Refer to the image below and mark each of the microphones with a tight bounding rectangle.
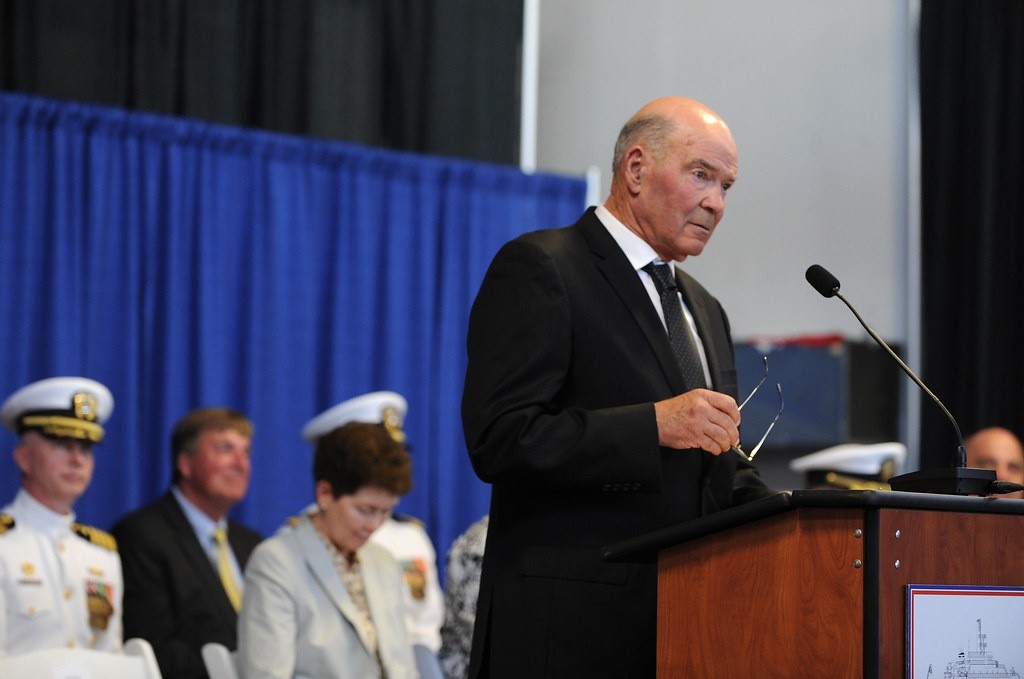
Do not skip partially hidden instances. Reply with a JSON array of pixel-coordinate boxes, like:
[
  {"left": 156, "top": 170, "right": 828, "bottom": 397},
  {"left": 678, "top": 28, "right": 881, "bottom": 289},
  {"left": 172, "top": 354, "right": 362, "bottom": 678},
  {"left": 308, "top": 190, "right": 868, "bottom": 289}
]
[{"left": 805, "top": 264, "right": 998, "bottom": 497}]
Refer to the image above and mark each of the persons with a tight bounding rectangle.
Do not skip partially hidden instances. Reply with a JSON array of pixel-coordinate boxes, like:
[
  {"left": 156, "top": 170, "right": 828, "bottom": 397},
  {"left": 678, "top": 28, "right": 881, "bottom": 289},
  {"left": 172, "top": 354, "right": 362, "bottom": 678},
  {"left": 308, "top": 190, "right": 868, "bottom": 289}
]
[
  {"left": 961, "top": 426, "right": 1024, "bottom": 499},
  {"left": 790, "top": 442, "right": 907, "bottom": 489},
  {"left": 104, "top": 408, "right": 267, "bottom": 679},
  {"left": 0, "top": 376, "right": 126, "bottom": 653},
  {"left": 237, "top": 390, "right": 488, "bottom": 679},
  {"left": 461, "top": 96, "right": 790, "bottom": 679}
]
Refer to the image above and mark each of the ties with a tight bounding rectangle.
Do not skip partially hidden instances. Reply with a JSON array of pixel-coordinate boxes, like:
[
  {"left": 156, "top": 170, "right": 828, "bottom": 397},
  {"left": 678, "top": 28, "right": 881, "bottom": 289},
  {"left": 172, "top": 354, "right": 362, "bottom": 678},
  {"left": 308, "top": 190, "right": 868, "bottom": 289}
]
[
  {"left": 210, "top": 531, "right": 239, "bottom": 612},
  {"left": 641, "top": 263, "right": 707, "bottom": 393}
]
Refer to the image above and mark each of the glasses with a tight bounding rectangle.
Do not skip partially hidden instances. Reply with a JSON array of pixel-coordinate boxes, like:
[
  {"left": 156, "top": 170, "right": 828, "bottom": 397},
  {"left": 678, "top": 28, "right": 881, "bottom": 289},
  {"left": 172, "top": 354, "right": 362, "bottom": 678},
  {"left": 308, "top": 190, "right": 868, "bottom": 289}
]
[{"left": 730, "top": 356, "right": 784, "bottom": 462}]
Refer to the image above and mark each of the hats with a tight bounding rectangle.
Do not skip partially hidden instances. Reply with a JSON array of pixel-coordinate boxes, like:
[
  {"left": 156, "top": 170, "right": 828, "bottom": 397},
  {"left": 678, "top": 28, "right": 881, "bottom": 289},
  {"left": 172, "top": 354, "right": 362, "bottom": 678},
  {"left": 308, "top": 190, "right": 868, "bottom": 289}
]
[
  {"left": 298, "top": 390, "right": 413, "bottom": 451},
  {"left": 3, "top": 376, "right": 115, "bottom": 445},
  {"left": 787, "top": 440, "right": 908, "bottom": 495}
]
